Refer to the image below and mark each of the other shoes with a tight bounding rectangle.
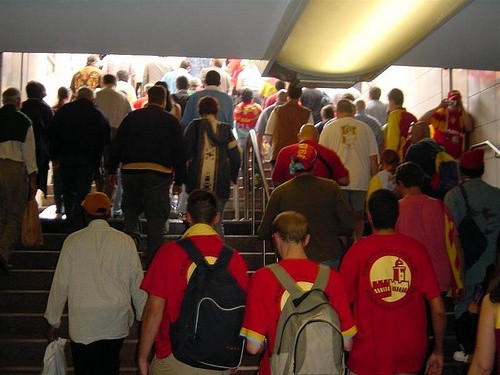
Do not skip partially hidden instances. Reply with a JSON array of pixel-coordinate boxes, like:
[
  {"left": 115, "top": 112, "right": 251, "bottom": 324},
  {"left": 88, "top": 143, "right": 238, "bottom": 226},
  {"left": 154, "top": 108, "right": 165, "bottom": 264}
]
[{"left": 453, "top": 350, "right": 473, "bottom": 363}]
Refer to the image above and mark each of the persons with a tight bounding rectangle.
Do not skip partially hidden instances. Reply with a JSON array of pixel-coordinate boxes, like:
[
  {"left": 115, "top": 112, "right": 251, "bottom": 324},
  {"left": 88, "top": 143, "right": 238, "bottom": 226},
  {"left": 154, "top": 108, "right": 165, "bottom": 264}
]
[
  {"left": 468, "top": 258, "right": 500, "bottom": 375},
  {"left": 139, "top": 191, "right": 249, "bottom": 375},
  {"left": 44, "top": 192, "right": 149, "bottom": 375},
  {"left": 444, "top": 148, "right": 500, "bottom": 285},
  {"left": 0, "top": 54, "right": 486, "bottom": 271},
  {"left": 393, "top": 161, "right": 463, "bottom": 293},
  {"left": 337, "top": 189, "right": 446, "bottom": 375},
  {"left": 238, "top": 210, "right": 357, "bottom": 375}
]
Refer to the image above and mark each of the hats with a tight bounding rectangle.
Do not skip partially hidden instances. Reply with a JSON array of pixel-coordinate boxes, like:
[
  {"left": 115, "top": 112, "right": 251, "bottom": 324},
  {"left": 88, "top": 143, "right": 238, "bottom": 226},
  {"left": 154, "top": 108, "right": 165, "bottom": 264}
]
[
  {"left": 458, "top": 147, "right": 485, "bottom": 178},
  {"left": 292, "top": 145, "right": 320, "bottom": 172},
  {"left": 80, "top": 191, "right": 112, "bottom": 216},
  {"left": 447, "top": 89, "right": 462, "bottom": 101}
]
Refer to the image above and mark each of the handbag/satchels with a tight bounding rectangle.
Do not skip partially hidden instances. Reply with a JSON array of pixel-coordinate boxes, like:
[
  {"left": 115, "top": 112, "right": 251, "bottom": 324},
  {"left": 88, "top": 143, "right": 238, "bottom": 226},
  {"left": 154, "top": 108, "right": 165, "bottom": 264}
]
[
  {"left": 456, "top": 215, "right": 488, "bottom": 270},
  {"left": 40, "top": 336, "right": 67, "bottom": 375},
  {"left": 20, "top": 198, "right": 44, "bottom": 247}
]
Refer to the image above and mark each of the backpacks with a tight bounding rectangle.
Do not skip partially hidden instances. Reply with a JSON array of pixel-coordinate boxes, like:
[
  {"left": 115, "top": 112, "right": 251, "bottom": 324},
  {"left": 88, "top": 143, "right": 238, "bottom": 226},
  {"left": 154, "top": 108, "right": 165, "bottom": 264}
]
[
  {"left": 168, "top": 237, "right": 248, "bottom": 372},
  {"left": 264, "top": 262, "right": 347, "bottom": 375}
]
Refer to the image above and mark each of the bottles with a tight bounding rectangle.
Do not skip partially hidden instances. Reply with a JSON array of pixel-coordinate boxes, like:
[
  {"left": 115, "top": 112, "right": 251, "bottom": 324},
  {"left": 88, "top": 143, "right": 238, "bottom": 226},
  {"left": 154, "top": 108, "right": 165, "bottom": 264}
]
[{"left": 169, "top": 192, "right": 179, "bottom": 214}]
